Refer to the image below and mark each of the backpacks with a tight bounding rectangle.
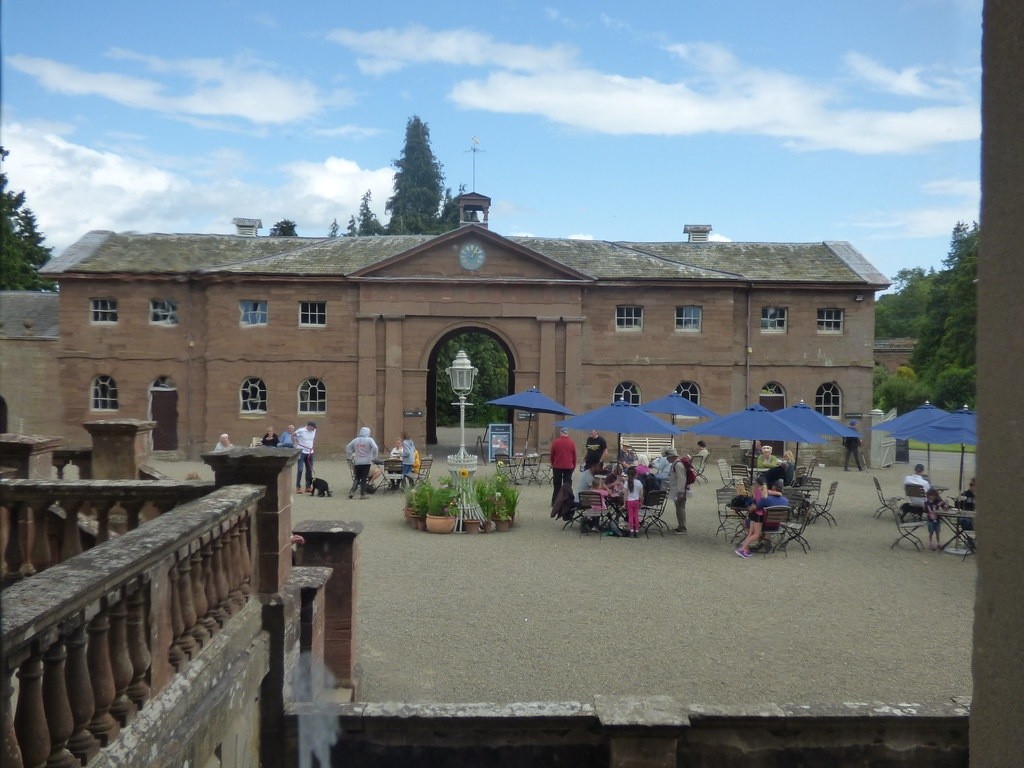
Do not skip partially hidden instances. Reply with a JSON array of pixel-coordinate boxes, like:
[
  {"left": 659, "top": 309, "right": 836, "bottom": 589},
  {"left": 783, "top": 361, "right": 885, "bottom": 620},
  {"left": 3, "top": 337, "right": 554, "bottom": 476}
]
[{"left": 673, "top": 454, "right": 697, "bottom": 491}]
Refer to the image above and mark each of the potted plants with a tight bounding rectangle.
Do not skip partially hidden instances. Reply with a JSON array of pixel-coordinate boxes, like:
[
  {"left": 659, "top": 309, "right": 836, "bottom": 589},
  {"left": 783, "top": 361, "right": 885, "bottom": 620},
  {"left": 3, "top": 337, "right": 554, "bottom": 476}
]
[
  {"left": 403, "top": 495, "right": 413, "bottom": 522},
  {"left": 484, "top": 501, "right": 493, "bottom": 532},
  {"left": 410, "top": 495, "right": 421, "bottom": 528},
  {"left": 426, "top": 489, "right": 457, "bottom": 533},
  {"left": 496, "top": 503, "right": 512, "bottom": 532},
  {"left": 504, "top": 489, "right": 521, "bottom": 528},
  {"left": 418, "top": 491, "right": 428, "bottom": 531},
  {"left": 464, "top": 508, "right": 481, "bottom": 535}
]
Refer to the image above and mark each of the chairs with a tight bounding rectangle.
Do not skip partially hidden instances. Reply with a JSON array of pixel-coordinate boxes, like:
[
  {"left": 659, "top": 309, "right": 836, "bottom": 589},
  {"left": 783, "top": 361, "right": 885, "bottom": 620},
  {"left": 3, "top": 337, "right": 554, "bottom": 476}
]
[
  {"left": 494, "top": 453, "right": 518, "bottom": 486},
  {"left": 874, "top": 476, "right": 904, "bottom": 518},
  {"left": 347, "top": 458, "right": 373, "bottom": 499},
  {"left": 889, "top": 501, "right": 927, "bottom": 552},
  {"left": 716, "top": 458, "right": 840, "bottom": 559},
  {"left": 382, "top": 458, "right": 404, "bottom": 494},
  {"left": 406, "top": 456, "right": 433, "bottom": 489},
  {"left": 561, "top": 452, "right": 714, "bottom": 540},
  {"left": 906, "top": 483, "right": 934, "bottom": 521},
  {"left": 529, "top": 451, "right": 554, "bottom": 485}
]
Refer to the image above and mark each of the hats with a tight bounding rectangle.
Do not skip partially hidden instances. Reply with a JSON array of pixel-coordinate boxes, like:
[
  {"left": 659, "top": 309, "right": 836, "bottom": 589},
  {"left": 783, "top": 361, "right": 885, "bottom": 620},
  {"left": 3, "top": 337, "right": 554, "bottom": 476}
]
[
  {"left": 560, "top": 427, "right": 568, "bottom": 434},
  {"left": 850, "top": 419, "right": 857, "bottom": 426},
  {"left": 663, "top": 450, "right": 679, "bottom": 457},
  {"left": 915, "top": 464, "right": 924, "bottom": 472},
  {"left": 620, "top": 442, "right": 631, "bottom": 447},
  {"left": 773, "top": 480, "right": 784, "bottom": 488},
  {"left": 756, "top": 476, "right": 767, "bottom": 486},
  {"left": 308, "top": 421, "right": 318, "bottom": 428}
]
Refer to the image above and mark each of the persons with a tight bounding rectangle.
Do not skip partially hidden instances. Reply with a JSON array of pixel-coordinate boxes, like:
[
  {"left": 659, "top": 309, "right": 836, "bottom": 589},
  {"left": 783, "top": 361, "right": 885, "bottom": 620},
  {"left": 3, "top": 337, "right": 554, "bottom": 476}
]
[
  {"left": 680, "top": 440, "right": 710, "bottom": 470},
  {"left": 261, "top": 421, "right": 317, "bottom": 494},
  {"left": 214, "top": 433, "right": 233, "bottom": 450},
  {"left": 548, "top": 428, "right": 687, "bottom": 539},
  {"left": 346, "top": 427, "right": 423, "bottom": 500},
  {"left": 923, "top": 480, "right": 946, "bottom": 552},
  {"left": 903, "top": 463, "right": 944, "bottom": 512},
  {"left": 841, "top": 418, "right": 870, "bottom": 473},
  {"left": 734, "top": 439, "right": 797, "bottom": 560},
  {"left": 954, "top": 475, "right": 977, "bottom": 545},
  {"left": 291, "top": 535, "right": 305, "bottom": 566}
]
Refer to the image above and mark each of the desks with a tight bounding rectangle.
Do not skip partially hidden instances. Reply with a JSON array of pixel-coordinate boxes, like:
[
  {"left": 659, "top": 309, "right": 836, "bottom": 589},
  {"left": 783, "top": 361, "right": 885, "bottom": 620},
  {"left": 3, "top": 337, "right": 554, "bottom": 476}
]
[
  {"left": 508, "top": 453, "right": 541, "bottom": 483},
  {"left": 940, "top": 507, "right": 976, "bottom": 559},
  {"left": 929, "top": 484, "right": 950, "bottom": 503},
  {"left": 747, "top": 467, "right": 771, "bottom": 485},
  {"left": 375, "top": 462, "right": 405, "bottom": 493}
]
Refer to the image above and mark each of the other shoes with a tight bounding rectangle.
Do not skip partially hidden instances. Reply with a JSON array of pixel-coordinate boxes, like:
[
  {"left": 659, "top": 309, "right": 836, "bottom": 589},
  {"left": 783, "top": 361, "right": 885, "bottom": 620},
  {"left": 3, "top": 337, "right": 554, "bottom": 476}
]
[
  {"left": 936, "top": 543, "right": 942, "bottom": 549},
  {"left": 928, "top": 544, "right": 936, "bottom": 550},
  {"left": 297, "top": 487, "right": 312, "bottom": 493},
  {"left": 629, "top": 532, "right": 635, "bottom": 538},
  {"left": 348, "top": 493, "right": 353, "bottom": 499},
  {"left": 858, "top": 467, "right": 863, "bottom": 471},
  {"left": 843, "top": 468, "right": 850, "bottom": 471},
  {"left": 636, "top": 532, "right": 639, "bottom": 538},
  {"left": 360, "top": 495, "right": 369, "bottom": 499}
]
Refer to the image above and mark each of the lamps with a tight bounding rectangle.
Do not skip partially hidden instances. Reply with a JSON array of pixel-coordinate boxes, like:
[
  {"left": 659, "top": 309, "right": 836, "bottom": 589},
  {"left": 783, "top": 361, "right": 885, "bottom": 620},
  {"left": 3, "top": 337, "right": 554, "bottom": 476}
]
[{"left": 856, "top": 293, "right": 865, "bottom": 301}]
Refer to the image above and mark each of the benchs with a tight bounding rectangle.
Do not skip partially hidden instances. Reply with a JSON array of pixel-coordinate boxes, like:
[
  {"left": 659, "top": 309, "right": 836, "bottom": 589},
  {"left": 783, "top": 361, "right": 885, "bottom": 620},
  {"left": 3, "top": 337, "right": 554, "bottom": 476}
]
[{"left": 619, "top": 436, "right": 676, "bottom": 463}]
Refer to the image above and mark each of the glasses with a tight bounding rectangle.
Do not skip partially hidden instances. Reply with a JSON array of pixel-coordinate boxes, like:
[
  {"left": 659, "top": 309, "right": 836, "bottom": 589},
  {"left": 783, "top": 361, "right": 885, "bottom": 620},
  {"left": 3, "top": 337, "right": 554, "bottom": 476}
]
[
  {"left": 969, "top": 483, "right": 975, "bottom": 487},
  {"left": 762, "top": 446, "right": 771, "bottom": 449}
]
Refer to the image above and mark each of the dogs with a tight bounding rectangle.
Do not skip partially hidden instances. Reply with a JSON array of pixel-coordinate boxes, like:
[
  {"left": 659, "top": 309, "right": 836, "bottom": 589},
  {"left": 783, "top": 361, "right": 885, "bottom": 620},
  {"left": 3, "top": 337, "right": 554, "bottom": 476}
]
[{"left": 307, "top": 475, "right": 332, "bottom": 497}]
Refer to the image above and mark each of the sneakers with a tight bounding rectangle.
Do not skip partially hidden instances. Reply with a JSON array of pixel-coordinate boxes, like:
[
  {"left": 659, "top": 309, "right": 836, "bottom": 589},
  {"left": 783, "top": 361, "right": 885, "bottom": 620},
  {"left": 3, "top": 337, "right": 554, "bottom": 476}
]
[
  {"left": 735, "top": 547, "right": 753, "bottom": 559},
  {"left": 671, "top": 527, "right": 688, "bottom": 535}
]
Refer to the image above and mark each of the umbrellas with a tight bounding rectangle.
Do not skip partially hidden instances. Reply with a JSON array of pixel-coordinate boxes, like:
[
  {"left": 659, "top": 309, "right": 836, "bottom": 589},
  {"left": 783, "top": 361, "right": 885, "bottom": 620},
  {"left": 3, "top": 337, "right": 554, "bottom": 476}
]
[
  {"left": 865, "top": 399, "right": 977, "bottom": 552},
  {"left": 484, "top": 384, "right": 577, "bottom": 480},
  {"left": 679, "top": 401, "right": 828, "bottom": 486},
  {"left": 766, "top": 397, "right": 866, "bottom": 472},
  {"left": 550, "top": 397, "right": 686, "bottom": 477},
  {"left": 634, "top": 390, "right": 722, "bottom": 450}
]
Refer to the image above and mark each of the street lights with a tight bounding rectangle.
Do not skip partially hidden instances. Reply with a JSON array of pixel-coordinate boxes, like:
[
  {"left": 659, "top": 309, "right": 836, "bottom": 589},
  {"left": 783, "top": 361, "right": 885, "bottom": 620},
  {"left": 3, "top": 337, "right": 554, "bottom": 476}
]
[{"left": 445, "top": 348, "right": 486, "bottom": 533}]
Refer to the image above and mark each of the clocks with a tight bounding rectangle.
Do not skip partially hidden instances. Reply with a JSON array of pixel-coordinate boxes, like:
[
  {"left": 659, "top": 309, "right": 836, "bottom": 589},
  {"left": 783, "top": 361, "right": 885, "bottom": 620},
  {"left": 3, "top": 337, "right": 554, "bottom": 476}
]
[{"left": 458, "top": 243, "right": 486, "bottom": 271}]
